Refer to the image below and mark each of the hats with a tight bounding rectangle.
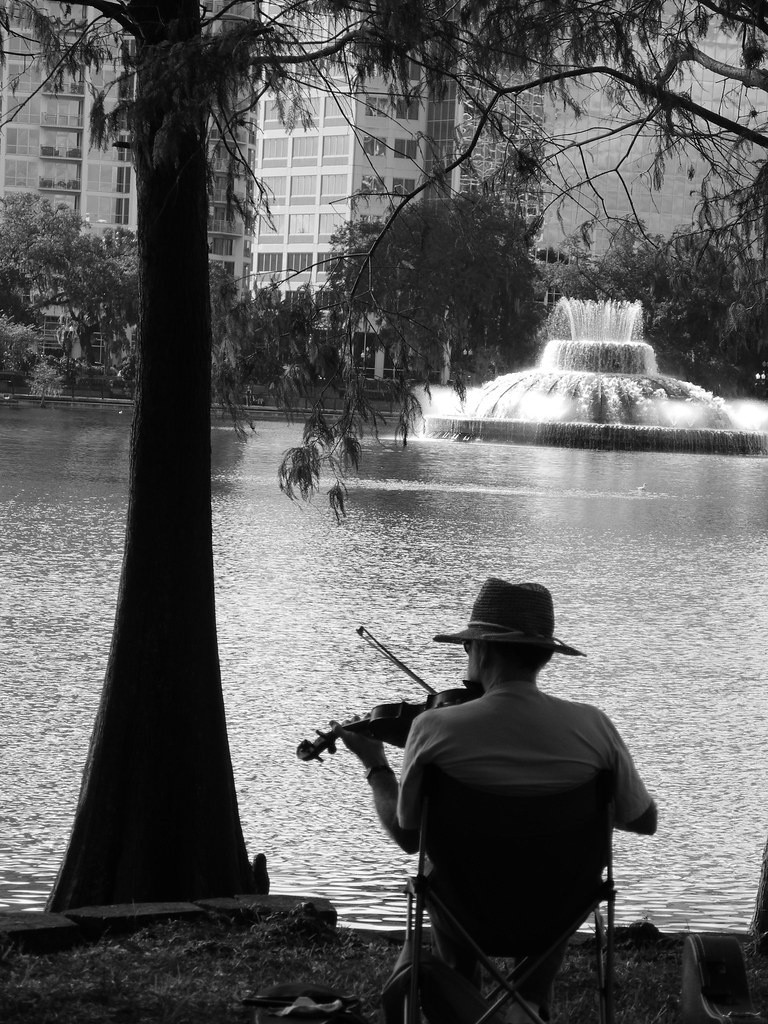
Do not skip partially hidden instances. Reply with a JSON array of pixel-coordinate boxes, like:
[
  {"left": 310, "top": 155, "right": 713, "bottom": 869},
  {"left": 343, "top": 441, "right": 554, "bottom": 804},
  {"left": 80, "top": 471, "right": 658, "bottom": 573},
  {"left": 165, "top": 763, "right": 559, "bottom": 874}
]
[{"left": 434, "top": 578, "right": 589, "bottom": 657}]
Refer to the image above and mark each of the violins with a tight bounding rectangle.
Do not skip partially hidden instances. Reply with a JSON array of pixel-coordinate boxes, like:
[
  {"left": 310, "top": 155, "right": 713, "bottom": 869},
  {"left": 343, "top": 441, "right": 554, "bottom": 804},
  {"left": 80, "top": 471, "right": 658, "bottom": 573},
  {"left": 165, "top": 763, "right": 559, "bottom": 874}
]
[{"left": 296, "top": 687, "right": 476, "bottom": 763}]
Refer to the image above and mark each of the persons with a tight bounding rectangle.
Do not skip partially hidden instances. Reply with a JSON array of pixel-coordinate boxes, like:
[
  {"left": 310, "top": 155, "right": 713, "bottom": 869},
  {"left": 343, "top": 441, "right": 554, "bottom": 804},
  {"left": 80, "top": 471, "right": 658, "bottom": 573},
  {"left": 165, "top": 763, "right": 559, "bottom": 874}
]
[{"left": 326, "top": 578, "right": 659, "bottom": 1003}]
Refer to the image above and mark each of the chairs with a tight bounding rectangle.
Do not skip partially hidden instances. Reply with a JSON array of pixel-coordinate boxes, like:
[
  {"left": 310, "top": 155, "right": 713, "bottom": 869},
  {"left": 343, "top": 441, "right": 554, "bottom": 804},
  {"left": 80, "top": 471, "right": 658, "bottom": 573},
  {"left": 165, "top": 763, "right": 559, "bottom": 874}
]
[{"left": 396, "top": 761, "right": 616, "bottom": 1024}]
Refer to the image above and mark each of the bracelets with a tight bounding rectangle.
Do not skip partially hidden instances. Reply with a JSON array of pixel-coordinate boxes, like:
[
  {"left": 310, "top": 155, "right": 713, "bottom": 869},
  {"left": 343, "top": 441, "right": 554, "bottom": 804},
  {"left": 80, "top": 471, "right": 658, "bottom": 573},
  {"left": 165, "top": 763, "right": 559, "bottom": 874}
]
[{"left": 361, "top": 764, "right": 397, "bottom": 780}]
